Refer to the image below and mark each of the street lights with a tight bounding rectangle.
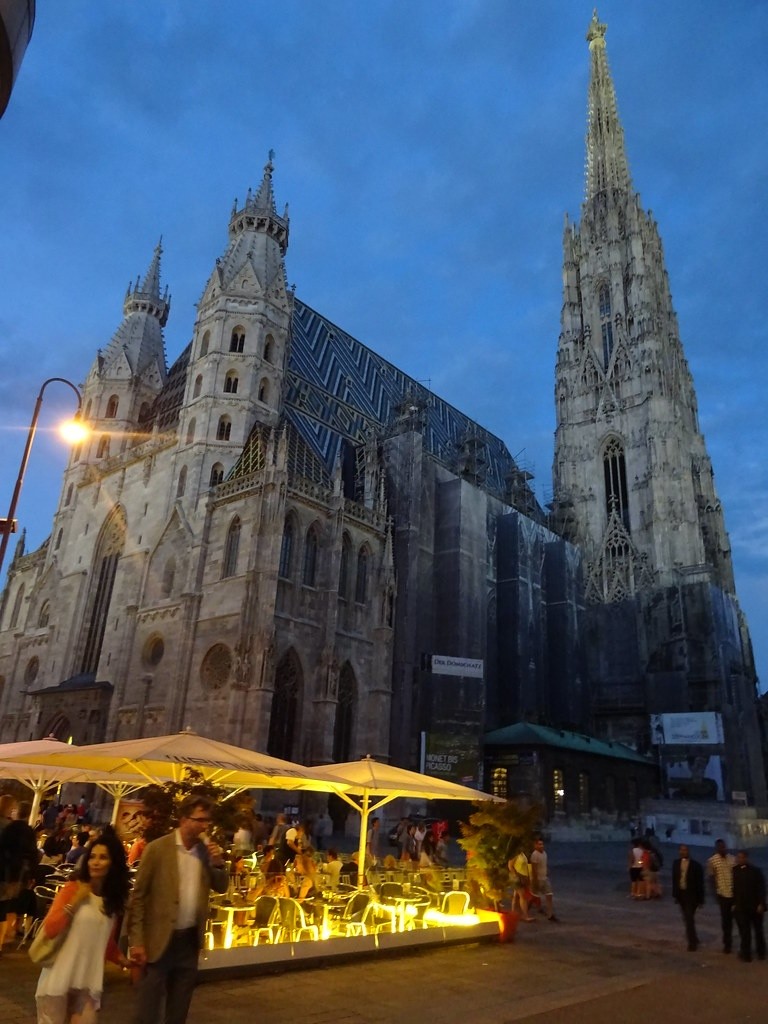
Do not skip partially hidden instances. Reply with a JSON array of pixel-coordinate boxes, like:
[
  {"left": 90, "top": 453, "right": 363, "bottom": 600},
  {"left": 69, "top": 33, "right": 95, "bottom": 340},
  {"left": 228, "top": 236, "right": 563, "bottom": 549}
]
[{"left": 0, "top": 377, "right": 86, "bottom": 572}]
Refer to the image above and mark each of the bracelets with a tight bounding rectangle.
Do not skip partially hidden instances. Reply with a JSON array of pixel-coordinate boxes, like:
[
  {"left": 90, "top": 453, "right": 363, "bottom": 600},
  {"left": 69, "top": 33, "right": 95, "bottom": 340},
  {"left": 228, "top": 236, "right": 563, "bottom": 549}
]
[{"left": 65, "top": 904, "right": 76, "bottom": 916}]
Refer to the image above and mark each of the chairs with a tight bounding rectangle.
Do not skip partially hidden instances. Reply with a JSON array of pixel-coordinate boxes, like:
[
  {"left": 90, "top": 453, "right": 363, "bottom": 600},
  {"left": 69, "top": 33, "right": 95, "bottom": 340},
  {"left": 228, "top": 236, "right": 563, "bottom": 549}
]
[
  {"left": 208, "top": 854, "right": 480, "bottom": 945},
  {"left": 17, "top": 859, "right": 136, "bottom": 949}
]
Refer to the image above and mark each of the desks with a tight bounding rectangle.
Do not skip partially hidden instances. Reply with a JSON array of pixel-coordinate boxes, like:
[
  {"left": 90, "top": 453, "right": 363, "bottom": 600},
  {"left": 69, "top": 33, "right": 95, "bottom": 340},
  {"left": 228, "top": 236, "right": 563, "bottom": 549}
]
[{"left": 13, "top": 850, "right": 493, "bottom": 956}]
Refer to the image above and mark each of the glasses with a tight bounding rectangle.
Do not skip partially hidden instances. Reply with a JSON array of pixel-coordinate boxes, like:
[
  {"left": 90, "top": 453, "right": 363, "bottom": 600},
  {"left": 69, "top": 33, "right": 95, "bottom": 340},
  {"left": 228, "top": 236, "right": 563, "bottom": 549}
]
[{"left": 186, "top": 816, "right": 217, "bottom": 825}]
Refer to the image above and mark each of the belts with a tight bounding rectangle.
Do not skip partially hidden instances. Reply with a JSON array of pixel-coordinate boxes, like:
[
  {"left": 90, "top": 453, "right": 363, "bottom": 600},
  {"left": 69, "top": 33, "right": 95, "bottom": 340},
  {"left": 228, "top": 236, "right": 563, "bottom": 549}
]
[{"left": 173, "top": 931, "right": 189, "bottom": 944}]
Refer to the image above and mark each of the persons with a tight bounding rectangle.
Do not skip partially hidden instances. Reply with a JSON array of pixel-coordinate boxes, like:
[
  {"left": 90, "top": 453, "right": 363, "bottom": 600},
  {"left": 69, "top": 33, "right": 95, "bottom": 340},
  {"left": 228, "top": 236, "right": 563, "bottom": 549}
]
[
  {"left": 681, "top": 757, "right": 718, "bottom": 799},
  {"left": 128, "top": 796, "right": 230, "bottom": 1024},
  {"left": 396, "top": 817, "right": 466, "bottom": 866},
  {"left": 673, "top": 845, "right": 705, "bottom": 951},
  {"left": 629, "top": 821, "right": 643, "bottom": 838},
  {"left": 324, "top": 849, "right": 342, "bottom": 886},
  {"left": 316, "top": 806, "right": 332, "bottom": 851},
  {"left": 367, "top": 818, "right": 380, "bottom": 856},
  {"left": 707, "top": 839, "right": 743, "bottom": 954},
  {"left": 628, "top": 838, "right": 663, "bottom": 899},
  {"left": 232, "top": 812, "right": 317, "bottom": 913},
  {"left": 340, "top": 851, "right": 401, "bottom": 885},
  {"left": 0, "top": 793, "right": 135, "bottom": 1024},
  {"left": 508, "top": 839, "right": 561, "bottom": 923},
  {"left": 732, "top": 850, "right": 768, "bottom": 960}
]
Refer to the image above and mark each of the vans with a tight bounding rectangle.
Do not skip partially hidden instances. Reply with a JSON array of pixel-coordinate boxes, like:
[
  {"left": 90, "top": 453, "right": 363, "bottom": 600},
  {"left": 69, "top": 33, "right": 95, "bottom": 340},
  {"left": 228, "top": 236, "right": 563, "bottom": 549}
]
[{"left": 388, "top": 817, "right": 440, "bottom": 845}]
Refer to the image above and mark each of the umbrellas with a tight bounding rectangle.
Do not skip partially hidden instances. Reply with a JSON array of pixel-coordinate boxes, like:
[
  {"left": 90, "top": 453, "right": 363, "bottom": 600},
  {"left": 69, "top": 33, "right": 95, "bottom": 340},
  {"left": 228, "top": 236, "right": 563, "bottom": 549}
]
[
  {"left": 309, "top": 753, "right": 506, "bottom": 890},
  {"left": 0, "top": 734, "right": 76, "bottom": 827},
  {"left": 0, "top": 726, "right": 373, "bottom": 800}
]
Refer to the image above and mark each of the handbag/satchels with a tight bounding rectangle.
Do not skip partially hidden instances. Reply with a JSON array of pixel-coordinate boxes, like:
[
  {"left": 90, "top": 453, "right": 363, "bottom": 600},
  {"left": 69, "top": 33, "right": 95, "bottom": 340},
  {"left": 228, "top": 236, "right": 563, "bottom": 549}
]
[
  {"left": 387, "top": 821, "right": 405, "bottom": 847},
  {"left": 27, "top": 879, "right": 83, "bottom": 968},
  {"left": 649, "top": 850, "right": 658, "bottom": 872}
]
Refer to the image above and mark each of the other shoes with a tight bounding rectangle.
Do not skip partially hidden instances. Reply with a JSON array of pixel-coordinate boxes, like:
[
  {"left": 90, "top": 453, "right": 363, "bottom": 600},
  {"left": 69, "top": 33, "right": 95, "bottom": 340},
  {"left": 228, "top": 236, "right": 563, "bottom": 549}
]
[
  {"left": 687, "top": 944, "right": 698, "bottom": 952},
  {"left": 548, "top": 914, "right": 558, "bottom": 921},
  {"left": 758, "top": 951, "right": 768, "bottom": 959},
  {"left": 521, "top": 916, "right": 537, "bottom": 922},
  {"left": 722, "top": 944, "right": 732, "bottom": 954},
  {"left": 739, "top": 950, "right": 752, "bottom": 960}
]
